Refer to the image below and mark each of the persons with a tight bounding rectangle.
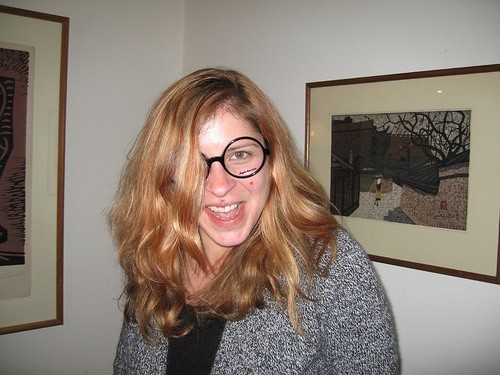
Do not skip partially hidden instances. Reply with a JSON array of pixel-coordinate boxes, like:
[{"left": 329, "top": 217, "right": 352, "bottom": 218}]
[{"left": 112, "top": 67, "right": 400, "bottom": 374}]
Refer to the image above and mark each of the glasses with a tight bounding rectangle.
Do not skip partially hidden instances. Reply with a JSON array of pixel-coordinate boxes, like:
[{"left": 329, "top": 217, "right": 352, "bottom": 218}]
[{"left": 168, "top": 136, "right": 271, "bottom": 186}]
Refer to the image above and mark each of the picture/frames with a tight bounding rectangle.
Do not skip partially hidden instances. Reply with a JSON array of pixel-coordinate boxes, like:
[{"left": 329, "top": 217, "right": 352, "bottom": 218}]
[
  {"left": 0, "top": 3, "right": 69, "bottom": 336},
  {"left": 302, "top": 63, "right": 500, "bottom": 285}
]
